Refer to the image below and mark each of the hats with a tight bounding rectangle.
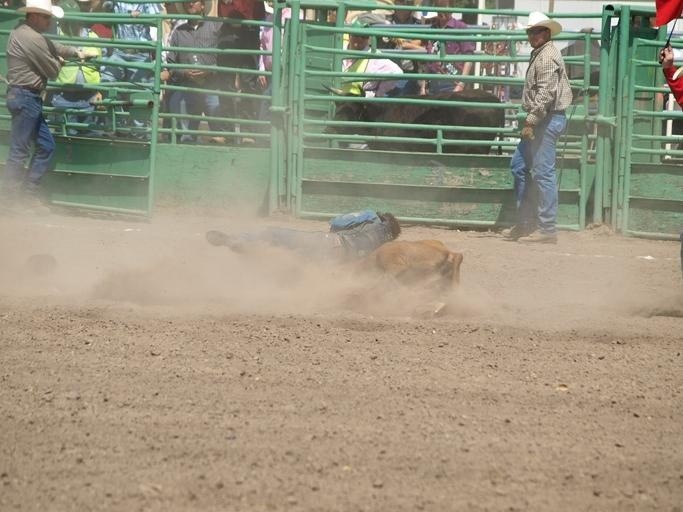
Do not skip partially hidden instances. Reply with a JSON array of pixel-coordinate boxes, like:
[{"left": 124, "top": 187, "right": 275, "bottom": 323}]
[
  {"left": 14, "top": 0, "right": 64, "bottom": 19},
  {"left": 517, "top": 11, "right": 562, "bottom": 36}
]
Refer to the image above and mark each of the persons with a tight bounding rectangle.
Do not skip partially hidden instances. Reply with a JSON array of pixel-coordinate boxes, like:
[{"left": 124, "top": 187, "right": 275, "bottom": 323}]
[
  {"left": 3, "top": 0, "right": 85, "bottom": 213},
  {"left": 502, "top": 9, "right": 574, "bottom": 244},
  {"left": 0, "top": 2, "right": 501, "bottom": 155},
  {"left": 654, "top": 45, "right": 683, "bottom": 113},
  {"left": 207, "top": 211, "right": 401, "bottom": 261}
]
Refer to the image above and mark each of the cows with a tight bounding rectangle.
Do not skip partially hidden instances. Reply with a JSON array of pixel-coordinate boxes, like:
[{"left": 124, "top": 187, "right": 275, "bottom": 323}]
[
  {"left": 204, "top": 230, "right": 465, "bottom": 313},
  {"left": 319, "top": 81, "right": 506, "bottom": 155}
]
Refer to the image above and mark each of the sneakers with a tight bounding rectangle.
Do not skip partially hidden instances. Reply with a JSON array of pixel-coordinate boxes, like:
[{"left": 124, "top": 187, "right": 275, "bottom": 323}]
[{"left": 500, "top": 219, "right": 557, "bottom": 246}]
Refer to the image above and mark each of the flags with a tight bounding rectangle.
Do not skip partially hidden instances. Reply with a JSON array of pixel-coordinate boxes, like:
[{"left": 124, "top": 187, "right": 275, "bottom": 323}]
[{"left": 654, "top": 0, "right": 683, "bottom": 27}]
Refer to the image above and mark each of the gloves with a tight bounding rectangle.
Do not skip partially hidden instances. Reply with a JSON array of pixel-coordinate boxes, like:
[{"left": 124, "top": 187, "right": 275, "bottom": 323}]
[{"left": 519, "top": 125, "right": 535, "bottom": 145}]
